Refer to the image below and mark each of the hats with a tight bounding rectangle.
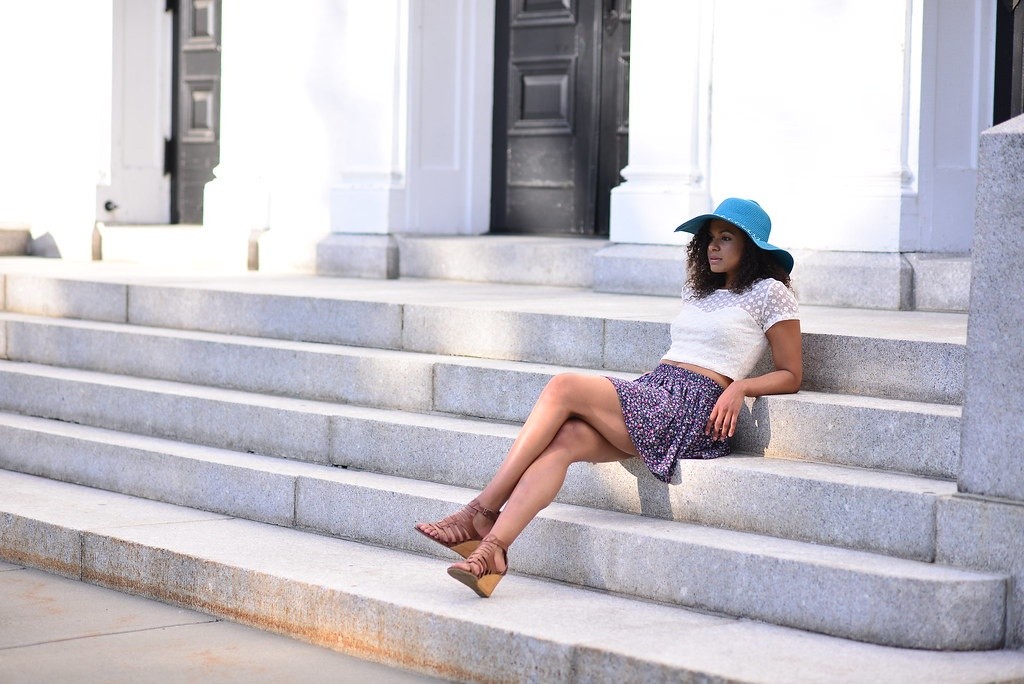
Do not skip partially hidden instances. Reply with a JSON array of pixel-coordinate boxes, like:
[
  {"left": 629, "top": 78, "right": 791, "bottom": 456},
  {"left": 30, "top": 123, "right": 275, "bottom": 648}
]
[{"left": 671, "top": 197, "right": 795, "bottom": 272}]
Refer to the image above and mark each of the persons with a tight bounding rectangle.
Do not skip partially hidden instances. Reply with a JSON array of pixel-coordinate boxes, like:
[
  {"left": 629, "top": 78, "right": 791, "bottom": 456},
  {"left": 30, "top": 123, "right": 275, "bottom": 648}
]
[{"left": 412, "top": 197, "right": 803, "bottom": 598}]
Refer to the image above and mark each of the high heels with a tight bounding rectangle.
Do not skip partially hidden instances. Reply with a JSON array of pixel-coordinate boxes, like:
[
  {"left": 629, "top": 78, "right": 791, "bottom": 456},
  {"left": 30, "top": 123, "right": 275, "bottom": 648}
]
[
  {"left": 413, "top": 497, "right": 501, "bottom": 560},
  {"left": 444, "top": 534, "right": 510, "bottom": 598}
]
